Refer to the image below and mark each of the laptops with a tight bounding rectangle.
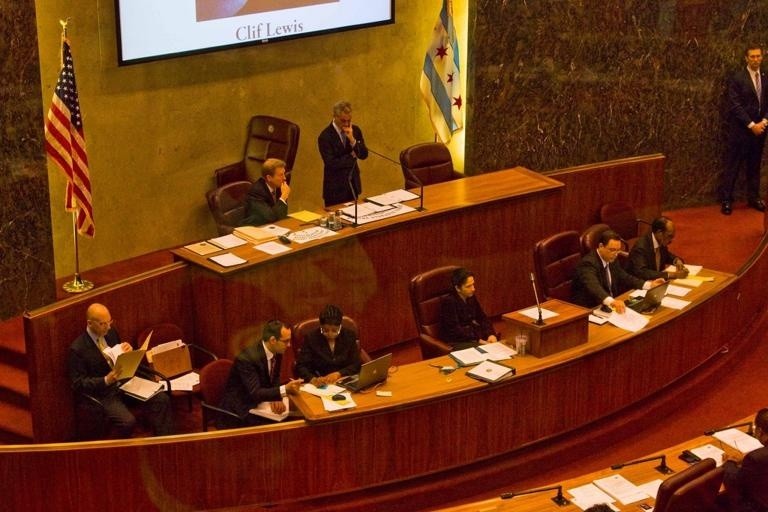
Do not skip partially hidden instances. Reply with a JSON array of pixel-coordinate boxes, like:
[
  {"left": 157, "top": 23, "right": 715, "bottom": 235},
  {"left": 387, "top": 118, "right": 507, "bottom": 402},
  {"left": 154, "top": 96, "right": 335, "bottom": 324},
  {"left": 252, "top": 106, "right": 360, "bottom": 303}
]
[
  {"left": 336, "top": 352, "right": 393, "bottom": 393},
  {"left": 625, "top": 280, "right": 670, "bottom": 312}
]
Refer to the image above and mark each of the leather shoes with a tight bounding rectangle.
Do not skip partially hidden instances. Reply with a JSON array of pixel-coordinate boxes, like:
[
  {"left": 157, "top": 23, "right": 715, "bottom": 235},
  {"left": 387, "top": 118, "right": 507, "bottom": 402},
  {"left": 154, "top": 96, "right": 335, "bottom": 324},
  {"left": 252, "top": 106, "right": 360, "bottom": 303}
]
[
  {"left": 721, "top": 200, "right": 732, "bottom": 215},
  {"left": 747, "top": 197, "right": 767, "bottom": 211}
]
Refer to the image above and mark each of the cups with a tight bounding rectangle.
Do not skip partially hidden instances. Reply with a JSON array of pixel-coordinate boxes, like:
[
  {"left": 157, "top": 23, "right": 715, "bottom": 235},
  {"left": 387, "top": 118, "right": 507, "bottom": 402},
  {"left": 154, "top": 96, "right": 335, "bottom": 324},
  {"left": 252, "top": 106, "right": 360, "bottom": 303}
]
[
  {"left": 328, "top": 214, "right": 336, "bottom": 229},
  {"left": 516, "top": 336, "right": 527, "bottom": 355},
  {"left": 320, "top": 215, "right": 328, "bottom": 226}
]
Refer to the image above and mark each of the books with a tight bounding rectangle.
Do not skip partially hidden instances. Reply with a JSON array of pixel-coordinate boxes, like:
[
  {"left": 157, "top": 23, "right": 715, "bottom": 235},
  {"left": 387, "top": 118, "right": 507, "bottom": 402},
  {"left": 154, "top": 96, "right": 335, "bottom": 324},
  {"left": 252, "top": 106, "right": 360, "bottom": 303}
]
[{"left": 250, "top": 395, "right": 290, "bottom": 423}]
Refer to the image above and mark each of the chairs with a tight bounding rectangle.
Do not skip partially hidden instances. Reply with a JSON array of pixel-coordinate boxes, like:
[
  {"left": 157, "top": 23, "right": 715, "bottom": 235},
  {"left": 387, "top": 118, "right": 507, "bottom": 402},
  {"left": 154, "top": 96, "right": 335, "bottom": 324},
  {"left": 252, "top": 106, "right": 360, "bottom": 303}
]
[
  {"left": 600, "top": 201, "right": 652, "bottom": 252},
  {"left": 533, "top": 230, "right": 584, "bottom": 303},
  {"left": 214, "top": 114, "right": 300, "bottom": 188},
  {"left": 653, "top": 457, "right": 726, "bottom": 512},
  {"left": 199, "top": 357, "right": 247, "bottom": 432},
  {"left": 68, "top": 334, "right": 159, "bottom": 435},
  {"left": 290, "top": 314, "right": 373, "bottom": 377},
  {"left": 400, "top": 142, "right": 469, "bottom": 190},
  {"left": 579, "top": 223, "right": 629, "bottom": 269},
  {"left": 204, "top": 180, "right": 253, "bottom": 237},
  {"left": 408, "top": 265, "right": 501, "bottom": 360},
  {"left": 137, "top": 322, "right": 218, "bottom": 411}
]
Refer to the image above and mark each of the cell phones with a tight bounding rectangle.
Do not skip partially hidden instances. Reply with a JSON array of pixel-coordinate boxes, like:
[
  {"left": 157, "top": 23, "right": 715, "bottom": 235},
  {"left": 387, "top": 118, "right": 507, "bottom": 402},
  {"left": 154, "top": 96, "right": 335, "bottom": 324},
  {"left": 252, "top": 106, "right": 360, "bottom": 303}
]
[{"left": 679, "top": 454, "right": 694, "bottom": 463}]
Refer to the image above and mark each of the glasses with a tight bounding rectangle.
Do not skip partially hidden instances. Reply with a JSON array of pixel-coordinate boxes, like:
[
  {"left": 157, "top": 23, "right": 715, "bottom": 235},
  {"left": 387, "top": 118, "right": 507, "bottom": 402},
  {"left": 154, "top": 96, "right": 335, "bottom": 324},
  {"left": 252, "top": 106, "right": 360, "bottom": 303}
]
[
  {"left": 719, "top": 440, "right": 743, "bottom": 455},
  {"left": 278, "top": 338, "right": 289, "bottom": 343},
  {"left": 320, "top": 324, "right": 342, "bottom": 336},
  {"left": 93, "top": 319, "right": 114, "bottom": 327}
]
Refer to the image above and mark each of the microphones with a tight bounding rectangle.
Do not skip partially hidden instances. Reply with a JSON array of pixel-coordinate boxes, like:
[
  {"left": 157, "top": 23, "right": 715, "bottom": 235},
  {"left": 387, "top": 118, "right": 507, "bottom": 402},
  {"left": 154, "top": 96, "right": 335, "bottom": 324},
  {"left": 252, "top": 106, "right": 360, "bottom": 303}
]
[
  {"left": 500, "top": 484, "right": 562, "bottom": 500},
  {"left": 530, "top": 272, "right": 543, "bottom": 325},
  {"left": 611, "top": 454, "right": 665, "bottom": 470},
  {"left": 704, "top": 422, "right": 753, "bottom": 436}
]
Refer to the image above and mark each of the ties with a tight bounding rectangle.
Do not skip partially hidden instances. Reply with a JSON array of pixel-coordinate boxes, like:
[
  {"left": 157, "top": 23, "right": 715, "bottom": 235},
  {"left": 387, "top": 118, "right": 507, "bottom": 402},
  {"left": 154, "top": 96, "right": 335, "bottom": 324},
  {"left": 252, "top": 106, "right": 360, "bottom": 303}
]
[
  {"left": 97, "top": 337, "right": 114, "bottom": 370},
  {"left": 341, "top": 131, "right": 346, "bottom": 148},
  {"left": 655, "top": 247, "right": 661, "bottom": 270},
  {"left": 269, "top": 357, "right": 277, "bottom": 383},
  {"left": 272, "top": 192, "right": 275, "bottom": 200},
  {"left": 604, "top": 265, "right": 610, "bottom": 286},
  {"left": 755, "top": 72, "right": 761, "bottom": 111}
]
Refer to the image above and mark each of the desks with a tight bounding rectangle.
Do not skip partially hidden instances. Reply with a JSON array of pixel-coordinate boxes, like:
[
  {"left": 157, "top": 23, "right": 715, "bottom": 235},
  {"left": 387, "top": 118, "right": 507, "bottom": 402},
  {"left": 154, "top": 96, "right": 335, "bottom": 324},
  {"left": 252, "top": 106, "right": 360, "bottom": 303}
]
[
  {"left": 430, "top": 411, "right": 759, "bottom": 512},
  {"left": 288, "top": 263, "right": 738, "bottom": 501},
  {"left": 168, "top": 165, "right": 566, "bottom": 384}
]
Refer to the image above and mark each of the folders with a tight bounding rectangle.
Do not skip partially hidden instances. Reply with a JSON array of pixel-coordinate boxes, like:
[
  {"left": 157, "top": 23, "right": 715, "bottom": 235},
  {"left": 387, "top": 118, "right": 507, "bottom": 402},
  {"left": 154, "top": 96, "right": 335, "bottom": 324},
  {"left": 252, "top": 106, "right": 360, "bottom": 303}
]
[
  {"left": 110, "top": 328, "right": 154, "bottom": 382},
  {"left": 465, "top": 359, "right": 516, "bottom": 385},
  {"left": 363, "top": 188, "right": 422, "bottom": 207},
  {"left": 448, "top": 341, "right": 518, "bottom": 368},
  {"left": 341, "top": 202, "right": 396, "bottom": 218},
  {"left": 118, "top": 376, "right": 165, "bottom": 400}
]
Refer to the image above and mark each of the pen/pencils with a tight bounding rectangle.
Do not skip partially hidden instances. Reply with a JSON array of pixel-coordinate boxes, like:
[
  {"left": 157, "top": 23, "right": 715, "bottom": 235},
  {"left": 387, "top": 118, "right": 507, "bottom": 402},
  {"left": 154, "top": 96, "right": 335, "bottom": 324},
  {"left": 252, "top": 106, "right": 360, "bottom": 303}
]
[
  {"left": 315, "top": 371, "right": 328, "bottom": 389},
  {"left": 428, "top": 362, "right": 443, "bottom": 369},
  {"left": 299, "top": 221, "right": 315, "bottom": 228},
  {"left": 289, "top": 378, "right": 302, "bottom": 386}
]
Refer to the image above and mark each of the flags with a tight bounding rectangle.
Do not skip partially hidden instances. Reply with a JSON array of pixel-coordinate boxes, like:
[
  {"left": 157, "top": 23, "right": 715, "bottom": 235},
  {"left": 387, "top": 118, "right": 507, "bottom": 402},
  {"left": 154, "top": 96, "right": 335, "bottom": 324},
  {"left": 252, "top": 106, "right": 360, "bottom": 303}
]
[
  {"left": 419, "top": 1, "right": 466, "bottom": 147},
  {"left": 46, "top": 16, "right": 98, "bottom": 238}
]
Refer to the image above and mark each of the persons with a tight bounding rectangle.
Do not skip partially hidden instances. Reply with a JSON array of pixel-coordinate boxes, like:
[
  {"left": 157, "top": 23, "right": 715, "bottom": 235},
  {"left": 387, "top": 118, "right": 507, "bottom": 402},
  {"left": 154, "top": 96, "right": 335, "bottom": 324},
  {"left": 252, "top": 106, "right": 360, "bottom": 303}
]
[
  {"left": 318, "top": 101, "right": 368, "bottom": 208},
  {"left": 569, "top": 230, "right": 666, "bottom": 315},
  {"left": 717, "top": 43, "right": 768, "bottom": 216},
  {"left": 719, "top": 408, "right": 767, "bottom": 512},
  {"left": 293, "top": 303, "right": 362, "bottom": 389},
  {"left": 624, "top": 215, "right": 688, "bottom": 280},
  {"left": 238, "top": 157, "right": 291, "bottom": 227},
  {"left": 437, "top": 266, "right": 498, "bottom": 351},
  {"left": 211, "top": 318, "right": 305, "bottom": 431},
  {"left": 65, "top": 301, "right": 171, "bottom": 439}
]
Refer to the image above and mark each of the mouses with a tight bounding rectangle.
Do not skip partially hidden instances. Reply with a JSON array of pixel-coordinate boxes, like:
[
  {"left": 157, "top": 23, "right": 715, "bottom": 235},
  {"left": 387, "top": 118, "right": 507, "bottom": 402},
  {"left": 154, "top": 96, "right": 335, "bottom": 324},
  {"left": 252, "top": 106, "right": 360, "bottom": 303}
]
[
  {"left": 332, "top": 394, "right": 346, "bottom": 401},
  {"left": 439, "top": 365, "right": 456, "bottom": 375},
  {"left": 601, "top": 305, "right": 612, "bottom": 313}
]
